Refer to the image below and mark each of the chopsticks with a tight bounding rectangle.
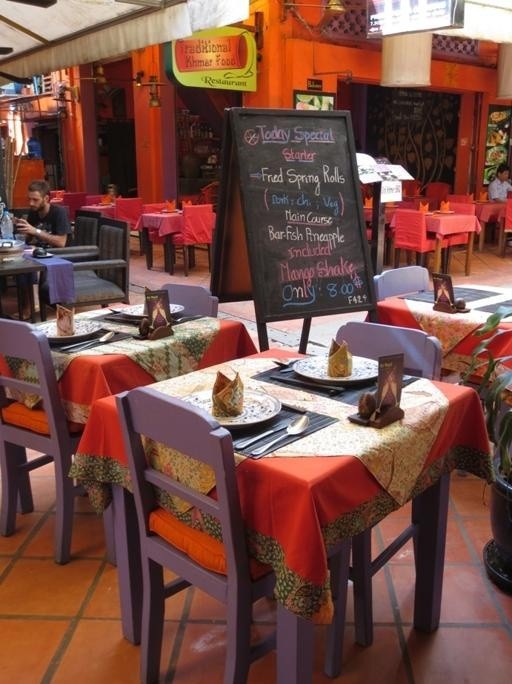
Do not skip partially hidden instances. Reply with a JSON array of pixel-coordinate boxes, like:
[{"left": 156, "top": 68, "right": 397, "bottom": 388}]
[
  {"left": 101, "top": 328, "right": 119, "bottom": 334},
  {"left": 282, "top": 402, "right": 307, "bottom": 415},
  {"left": 271, "top": 375, "right": 345, "bottom": 391},
  {"left": 104, "top": 316, "right": 142, "bottom": 323}
]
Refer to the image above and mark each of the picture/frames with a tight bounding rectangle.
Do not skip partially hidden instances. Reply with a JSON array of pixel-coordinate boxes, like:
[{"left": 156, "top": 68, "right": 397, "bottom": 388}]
[{"left": 293, "top": 89, "right": 336, "bottom": 111}]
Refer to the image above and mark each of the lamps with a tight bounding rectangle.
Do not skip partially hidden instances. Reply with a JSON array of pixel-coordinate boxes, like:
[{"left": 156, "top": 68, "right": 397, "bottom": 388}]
[
  {"left": 282, "top": 0, "right": 346, "bottom": 22},
  {"left": 136, "top": 46, "right": 169, "bottom": 107},
  {"left": 52, "top": 77, "right": 98, "bottom": 119}
]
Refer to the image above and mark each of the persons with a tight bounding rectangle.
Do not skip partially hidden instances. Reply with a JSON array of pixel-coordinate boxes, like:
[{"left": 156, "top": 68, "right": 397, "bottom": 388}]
[
  {"left": 12, "top": 180, "right": 75, "bottom": 320},
  {"left": 0, "top": 193, "right": 15, "bottom": 239},
  {"left": 488, "top": 163, "right": 512, "bottom": 248}
]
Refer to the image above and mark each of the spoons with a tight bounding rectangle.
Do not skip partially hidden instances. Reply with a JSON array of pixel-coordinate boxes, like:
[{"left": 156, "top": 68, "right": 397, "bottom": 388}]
[
  {"left": 272, "top": 358, "right": 303, "bottom": 367},
  {"left": 251, "top": 415, "right": 309, "bottom": 455},
  {"left": 108, "top": 308, "right": 122, "bottom": 313},
  {"left": 67, "top": 331, "right": 115, "bottom": 353}
]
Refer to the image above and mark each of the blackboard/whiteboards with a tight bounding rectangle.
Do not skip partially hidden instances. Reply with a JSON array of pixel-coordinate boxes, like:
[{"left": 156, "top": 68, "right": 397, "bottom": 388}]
[{"left": 229, "top": 107, "right": 377, "bottom": 322}]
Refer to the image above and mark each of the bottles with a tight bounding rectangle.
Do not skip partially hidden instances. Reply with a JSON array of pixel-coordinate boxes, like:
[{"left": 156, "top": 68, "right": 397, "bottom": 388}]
[{"left": 0, "top": 211, "right": 13, "bottom": 240}]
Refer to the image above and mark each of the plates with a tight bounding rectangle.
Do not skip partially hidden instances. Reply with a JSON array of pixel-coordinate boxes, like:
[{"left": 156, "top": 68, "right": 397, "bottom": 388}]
[
  {"left": 119, "top": 304, "right": 185, "bottom": 316},
  {"left": 35, "top": 318, "right": 103, "bottom": 345},
  {"left": 295, "top": 354, "right": 379, "bottom": 386},
  {"left": 31, "top": 252, "right": 54, "bottom": 258},
  {"left": 180, "top": 388, "right": 281, "bottom": 429},
  {"left": 52, "top": 197, "right": 64, "bottom": 200},
  {"left": 363, "top": 204, "right": 454, "bottom": 215},
  {"left": 99, "top": 201, "right": 117, "bottom": 206},
  {"left": 469, "top": 199, "right": 488, "bottom": 203},
  {"left": 152, "top": 209, "right": 184, "bottom": 214}
]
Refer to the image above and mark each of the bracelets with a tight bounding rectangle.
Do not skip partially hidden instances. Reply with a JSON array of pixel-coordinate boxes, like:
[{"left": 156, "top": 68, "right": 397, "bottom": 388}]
[{"left": 36, "top": 228, "right": 41, "bottom": 237}]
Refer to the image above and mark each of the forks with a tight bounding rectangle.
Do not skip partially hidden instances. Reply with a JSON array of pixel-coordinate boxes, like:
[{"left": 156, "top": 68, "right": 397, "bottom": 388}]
[{"left": 170, "top": 315, "right": 203, "bottom": 322}]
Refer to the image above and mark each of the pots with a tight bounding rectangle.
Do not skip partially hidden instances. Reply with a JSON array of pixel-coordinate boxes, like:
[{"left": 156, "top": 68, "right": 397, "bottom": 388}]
[{"left": 1, "top": 239, "right": 25, "bottom": 262}]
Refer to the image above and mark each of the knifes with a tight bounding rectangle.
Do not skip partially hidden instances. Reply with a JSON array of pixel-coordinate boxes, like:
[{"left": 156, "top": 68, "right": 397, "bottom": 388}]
[
  {"left": 233, "top": 415, "right": 300, "bottom": 449},
  {"left": 60, "top": 331, "right": 119, "bottom": 351},
  {"left": 279, "top": 367, "right": 294, "bottom": 373}
]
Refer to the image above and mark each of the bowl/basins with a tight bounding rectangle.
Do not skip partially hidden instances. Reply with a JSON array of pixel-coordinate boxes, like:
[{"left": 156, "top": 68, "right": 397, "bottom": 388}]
[{"left": 33, "top": 247, "right": 46, "bottom": 256}]
